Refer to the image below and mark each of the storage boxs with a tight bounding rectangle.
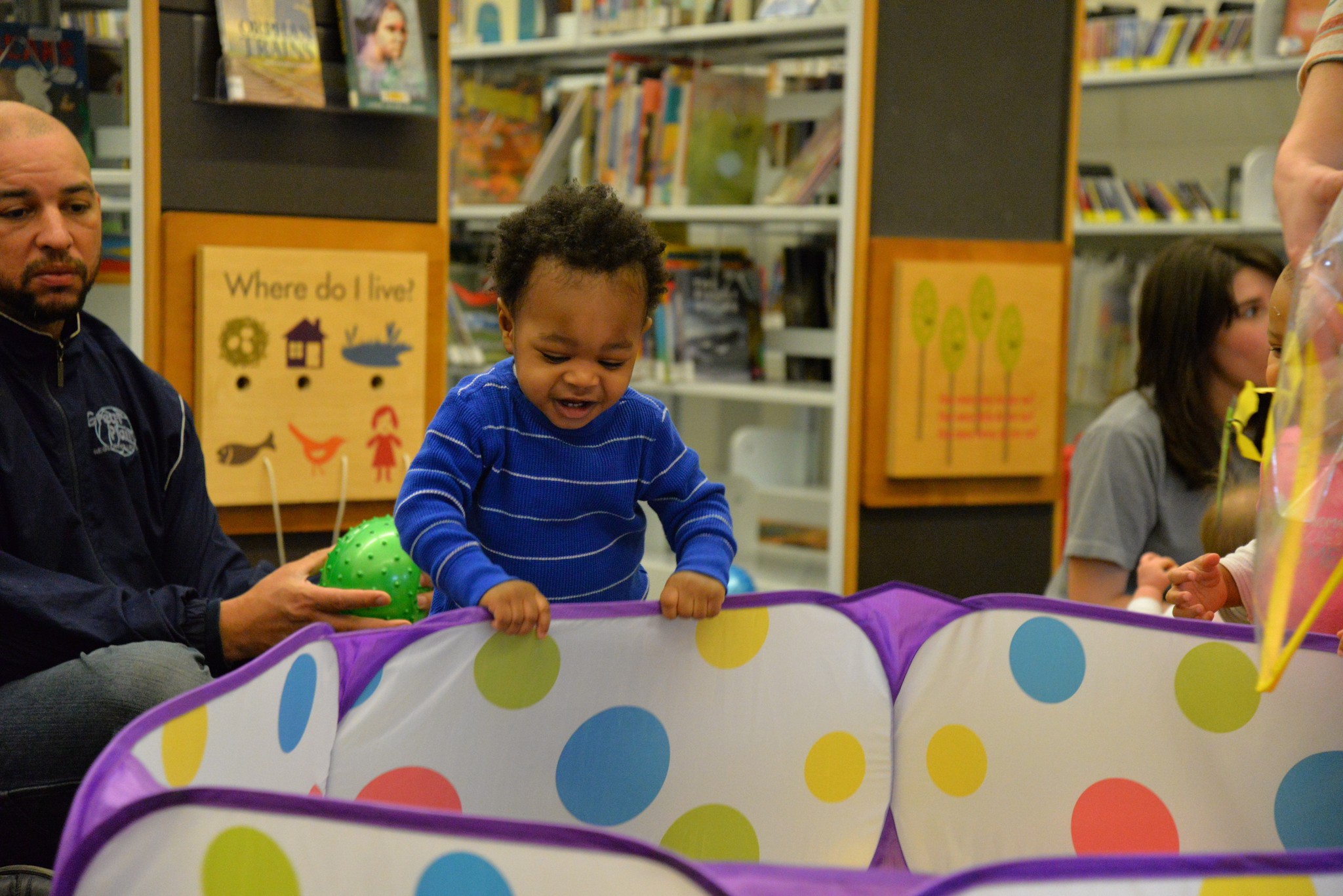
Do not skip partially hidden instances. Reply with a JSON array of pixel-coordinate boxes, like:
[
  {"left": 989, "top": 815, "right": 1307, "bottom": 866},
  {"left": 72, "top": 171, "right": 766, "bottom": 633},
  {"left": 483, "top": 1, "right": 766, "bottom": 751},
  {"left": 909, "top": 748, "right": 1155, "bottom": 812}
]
[{"left": 723, "top": 424, "right": 828, "bottom": 562}]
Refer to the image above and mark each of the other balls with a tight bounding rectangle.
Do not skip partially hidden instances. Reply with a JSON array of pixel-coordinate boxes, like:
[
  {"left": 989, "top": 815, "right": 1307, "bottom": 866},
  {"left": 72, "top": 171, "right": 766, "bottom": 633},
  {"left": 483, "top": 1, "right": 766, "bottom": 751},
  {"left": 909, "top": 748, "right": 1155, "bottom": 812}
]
[{"left": 319, "top": 515, "right": 431, "bottom": 627}]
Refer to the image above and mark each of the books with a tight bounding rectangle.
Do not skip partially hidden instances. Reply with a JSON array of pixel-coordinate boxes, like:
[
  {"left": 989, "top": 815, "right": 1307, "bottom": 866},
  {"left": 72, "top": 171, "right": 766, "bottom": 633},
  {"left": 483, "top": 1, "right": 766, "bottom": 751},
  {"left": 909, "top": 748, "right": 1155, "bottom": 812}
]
[
  {"left": 448, "top": 0, "right": 843, "bottom": 383},
  {"left": 61, "top": 9, "right": 128, "bottom": 42},
  {"left": 216, "top": 0, "right": 325, "bottom": 108},
  {"left": 0, "top": 22, "right": 95, "bottom": 167},
  {"left": 92, "top": 158, "right": 130, "bottom": 274},
  {"left": 335, "top": 0, "right": 432, "bottom": 111},
  {"left": 1075, "top": 175, "right": 1240, "bottom": 225},
  {"left": 1084, "top": 14, "right": 1251, "bottom": 74}
]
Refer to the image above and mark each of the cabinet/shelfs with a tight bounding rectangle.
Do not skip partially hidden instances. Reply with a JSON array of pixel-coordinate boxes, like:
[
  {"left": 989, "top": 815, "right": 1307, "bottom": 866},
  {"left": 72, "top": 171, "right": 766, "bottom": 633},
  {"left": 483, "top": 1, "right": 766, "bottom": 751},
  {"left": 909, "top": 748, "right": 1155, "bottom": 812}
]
[
  {"left": 1051, "top": 0, "right": 1330, "bottom": 492},
  {"left": 422, "top": 0, "right": 1084, "bottom": 602},
  {"left": 0, "top": 0, "right": 451, "bottom": 539}
]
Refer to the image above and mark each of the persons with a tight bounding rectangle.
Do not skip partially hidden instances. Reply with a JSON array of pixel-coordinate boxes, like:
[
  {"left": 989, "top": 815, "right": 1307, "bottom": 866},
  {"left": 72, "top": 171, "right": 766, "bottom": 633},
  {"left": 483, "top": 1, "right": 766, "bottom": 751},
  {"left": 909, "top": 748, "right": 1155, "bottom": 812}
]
[
  {"left": 1166, "top": 258, "right": 1343, "bottom": 625},
  {"left": 353, "top": 0, "right": 425, "bottom": 106},
  {"left": 1042, "top": 234, "right": 1287, "bottom": 609},
  {"left": 393, "top": 176, "right": 737, "bottom": 640},
  {"left": 0, "top": 103, "right": 437, "bottom": 866},
  {"left": 1127, "top": 483, "right": 1259, "bottom": 625}
]
